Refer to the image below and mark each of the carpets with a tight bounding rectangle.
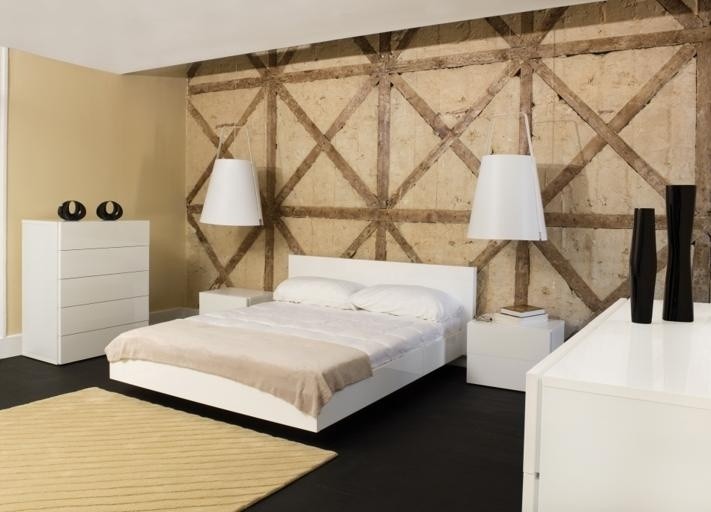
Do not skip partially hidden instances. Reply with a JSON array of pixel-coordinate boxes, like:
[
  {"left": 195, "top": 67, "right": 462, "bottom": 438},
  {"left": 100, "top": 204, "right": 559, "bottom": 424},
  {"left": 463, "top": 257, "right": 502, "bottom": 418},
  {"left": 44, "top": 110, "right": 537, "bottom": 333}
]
[{"left": 1, "top": 382, "right": 340, "bottom": 510}]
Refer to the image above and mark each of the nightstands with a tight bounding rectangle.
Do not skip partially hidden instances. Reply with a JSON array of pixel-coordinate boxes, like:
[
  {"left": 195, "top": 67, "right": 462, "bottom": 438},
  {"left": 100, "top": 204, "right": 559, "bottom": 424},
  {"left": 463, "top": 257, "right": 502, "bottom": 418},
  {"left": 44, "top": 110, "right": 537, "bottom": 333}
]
[
  {"left": 198, "top": 287, "right": 273, "bottom": 316},
  {"left": 465, "top": 312, "right": 566, "bottom": 392}
]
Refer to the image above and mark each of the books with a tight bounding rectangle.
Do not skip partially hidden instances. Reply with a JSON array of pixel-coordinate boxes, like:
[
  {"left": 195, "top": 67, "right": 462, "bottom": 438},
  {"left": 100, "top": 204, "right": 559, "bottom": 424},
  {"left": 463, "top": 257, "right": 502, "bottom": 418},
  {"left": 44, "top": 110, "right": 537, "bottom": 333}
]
[{"left": 501, "top": 303, "right": 547, "bottom": 318}]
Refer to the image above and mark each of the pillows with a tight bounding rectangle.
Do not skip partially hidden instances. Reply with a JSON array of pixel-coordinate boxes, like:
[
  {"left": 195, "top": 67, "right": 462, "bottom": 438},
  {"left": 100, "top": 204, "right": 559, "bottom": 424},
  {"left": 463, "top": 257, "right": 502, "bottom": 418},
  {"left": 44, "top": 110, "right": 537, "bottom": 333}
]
[{"left": 272, "top": 274, "right": 465, "bottom": 324}]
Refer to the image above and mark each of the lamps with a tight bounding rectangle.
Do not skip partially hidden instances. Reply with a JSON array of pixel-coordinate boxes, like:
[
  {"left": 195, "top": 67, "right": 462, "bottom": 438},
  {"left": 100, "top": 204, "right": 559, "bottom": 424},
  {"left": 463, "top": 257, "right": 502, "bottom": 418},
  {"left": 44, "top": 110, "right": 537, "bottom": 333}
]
[
  {"left": 197, "top": 55, "right": 266, "bottom": 229},
  {"left": 464, "top": 13, "right": 550, "bottom": 244}
]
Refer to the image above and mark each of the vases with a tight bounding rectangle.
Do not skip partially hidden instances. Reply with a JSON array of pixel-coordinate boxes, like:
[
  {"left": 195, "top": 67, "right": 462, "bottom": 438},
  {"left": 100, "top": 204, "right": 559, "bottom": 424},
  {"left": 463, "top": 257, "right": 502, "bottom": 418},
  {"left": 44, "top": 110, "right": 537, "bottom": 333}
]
[{"left": 628, "top": 183, "right": 698, "bottom": 325}]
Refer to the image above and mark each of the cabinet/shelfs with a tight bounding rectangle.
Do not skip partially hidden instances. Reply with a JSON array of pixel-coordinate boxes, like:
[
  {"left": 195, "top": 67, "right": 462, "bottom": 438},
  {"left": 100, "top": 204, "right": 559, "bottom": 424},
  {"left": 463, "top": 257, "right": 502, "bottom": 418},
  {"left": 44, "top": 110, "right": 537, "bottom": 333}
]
[
  {"left": 520, "top": 295, "right": 710, "bottom": 512},
  {"left": 18, "top": 216, "right": 153, "bottom": 367}
]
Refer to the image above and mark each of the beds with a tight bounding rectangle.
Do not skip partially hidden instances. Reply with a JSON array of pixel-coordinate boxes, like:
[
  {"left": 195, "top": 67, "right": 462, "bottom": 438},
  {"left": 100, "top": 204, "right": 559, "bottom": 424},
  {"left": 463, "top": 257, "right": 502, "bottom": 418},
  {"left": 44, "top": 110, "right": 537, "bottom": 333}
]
[{"left": 108, "top": 252, "right": 481, "bottom": 435}]
